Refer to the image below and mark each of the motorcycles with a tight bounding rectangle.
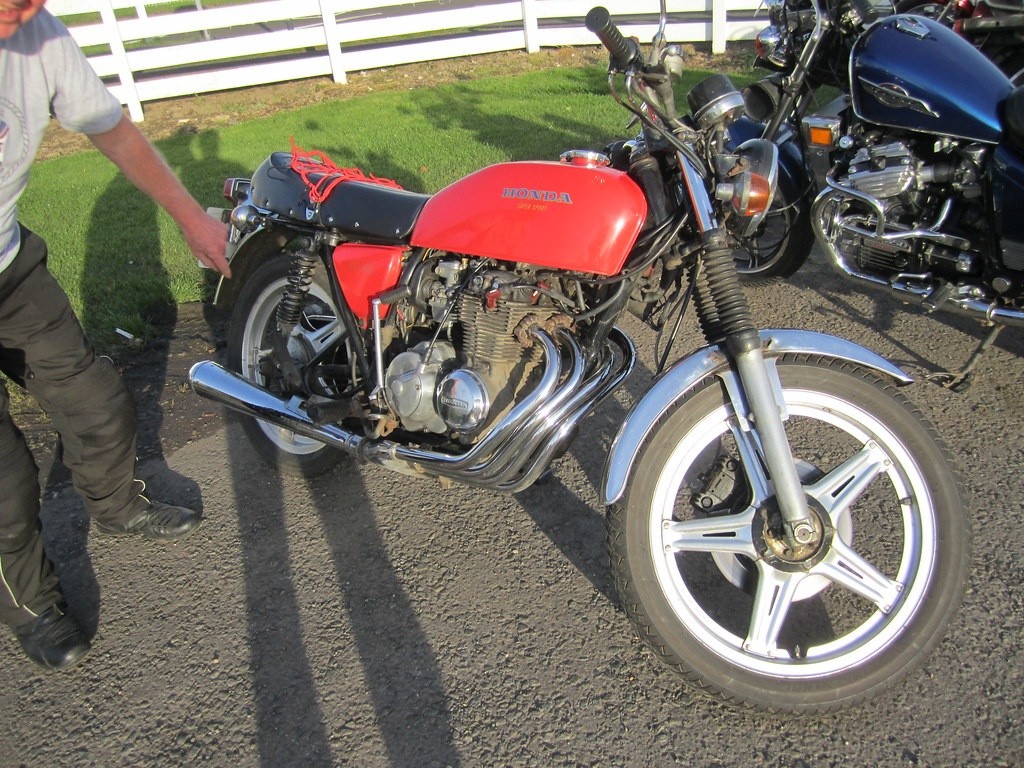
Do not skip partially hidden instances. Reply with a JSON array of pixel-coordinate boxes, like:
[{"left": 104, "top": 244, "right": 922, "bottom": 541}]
[
  {"left": 187, "top": 0, "right": 973, "bottom": 717},
  {"left": 680, "top": 0, "right": 1024, "bottom": 395}
]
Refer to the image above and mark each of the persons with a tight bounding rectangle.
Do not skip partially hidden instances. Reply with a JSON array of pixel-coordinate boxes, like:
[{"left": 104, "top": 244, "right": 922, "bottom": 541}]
[{"left": 1, "top": 1, "right": 232, "bottom": 669}]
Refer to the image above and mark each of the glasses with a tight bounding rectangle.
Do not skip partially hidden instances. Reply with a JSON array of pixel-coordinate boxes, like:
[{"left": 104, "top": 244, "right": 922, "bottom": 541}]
[{"left": 0, "top": 6, "right": 23, "bottom": 23}]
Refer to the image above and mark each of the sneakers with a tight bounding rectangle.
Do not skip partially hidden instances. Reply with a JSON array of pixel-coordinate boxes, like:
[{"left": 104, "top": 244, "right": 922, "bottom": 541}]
[
  {"left": 12, "top": 602, "right": 93, "bottom": 671},
  {"left": 95, "top": 501, "right": 200, "bottom": 542}
]
